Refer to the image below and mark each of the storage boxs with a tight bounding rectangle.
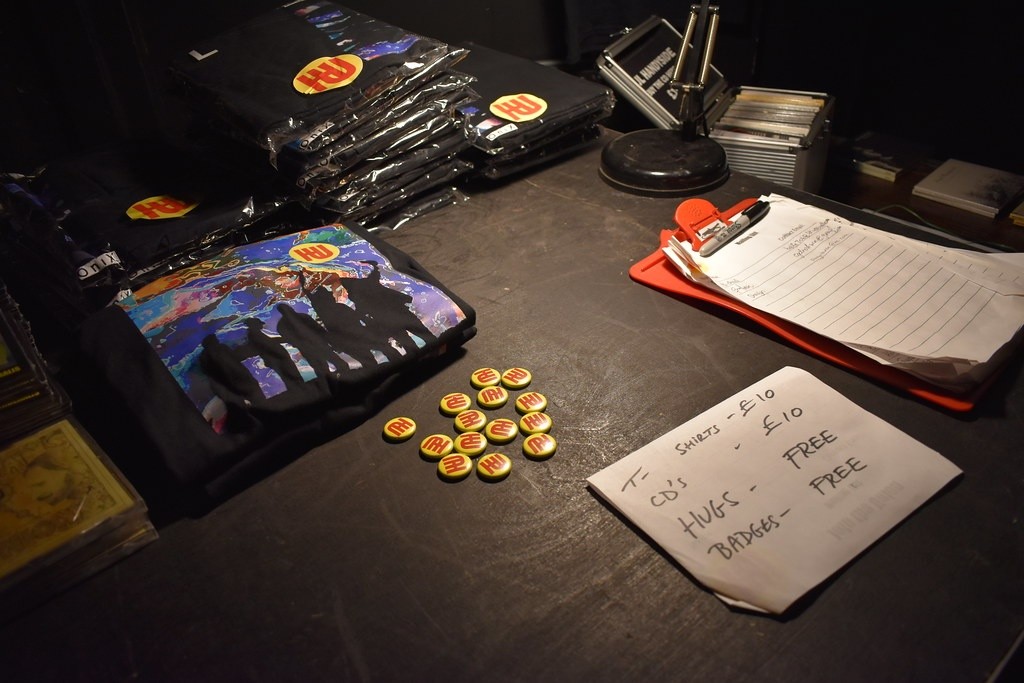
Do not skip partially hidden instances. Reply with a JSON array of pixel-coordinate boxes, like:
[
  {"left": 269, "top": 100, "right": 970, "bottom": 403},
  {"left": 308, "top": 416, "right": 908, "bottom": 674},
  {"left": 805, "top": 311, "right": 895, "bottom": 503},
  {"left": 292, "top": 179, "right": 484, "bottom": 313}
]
[{"left": 596, "top": 15, "right": 838, "bottom": 196}]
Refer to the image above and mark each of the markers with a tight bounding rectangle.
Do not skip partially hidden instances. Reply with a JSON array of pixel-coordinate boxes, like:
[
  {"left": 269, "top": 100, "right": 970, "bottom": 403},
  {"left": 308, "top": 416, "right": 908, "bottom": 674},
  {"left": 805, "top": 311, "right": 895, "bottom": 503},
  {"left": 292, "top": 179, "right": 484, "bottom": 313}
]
[{"left": 699, "top": 200, "right": 770, "bottom": 256}]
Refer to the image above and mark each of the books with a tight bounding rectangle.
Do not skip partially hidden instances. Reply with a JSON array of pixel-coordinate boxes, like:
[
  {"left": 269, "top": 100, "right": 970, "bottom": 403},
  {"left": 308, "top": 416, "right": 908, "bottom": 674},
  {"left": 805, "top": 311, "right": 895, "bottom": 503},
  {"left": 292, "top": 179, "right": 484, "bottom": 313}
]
[
  {"left": 710, "top": 89, "right": 825, "bottom": 144},
  {"left": 911, "top": 158, "right": 1024, "bottom": 219},
  {"left": 1009, "top": 202, "right": 1024, "bottom": 226},
  {"left": 0, "top": 420, "right": 159, "bottom": 627},
  {"left": 844, "top": 130, "right": 925, "bottom": 184}
]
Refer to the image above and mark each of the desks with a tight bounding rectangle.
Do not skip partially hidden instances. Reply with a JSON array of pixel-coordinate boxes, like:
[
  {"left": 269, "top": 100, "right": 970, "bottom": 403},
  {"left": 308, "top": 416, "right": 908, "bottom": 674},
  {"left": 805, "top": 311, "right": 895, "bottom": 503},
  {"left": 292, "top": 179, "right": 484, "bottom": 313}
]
[{"left": 1, "top": 88, "right": 1023, "bottom": 683}]
